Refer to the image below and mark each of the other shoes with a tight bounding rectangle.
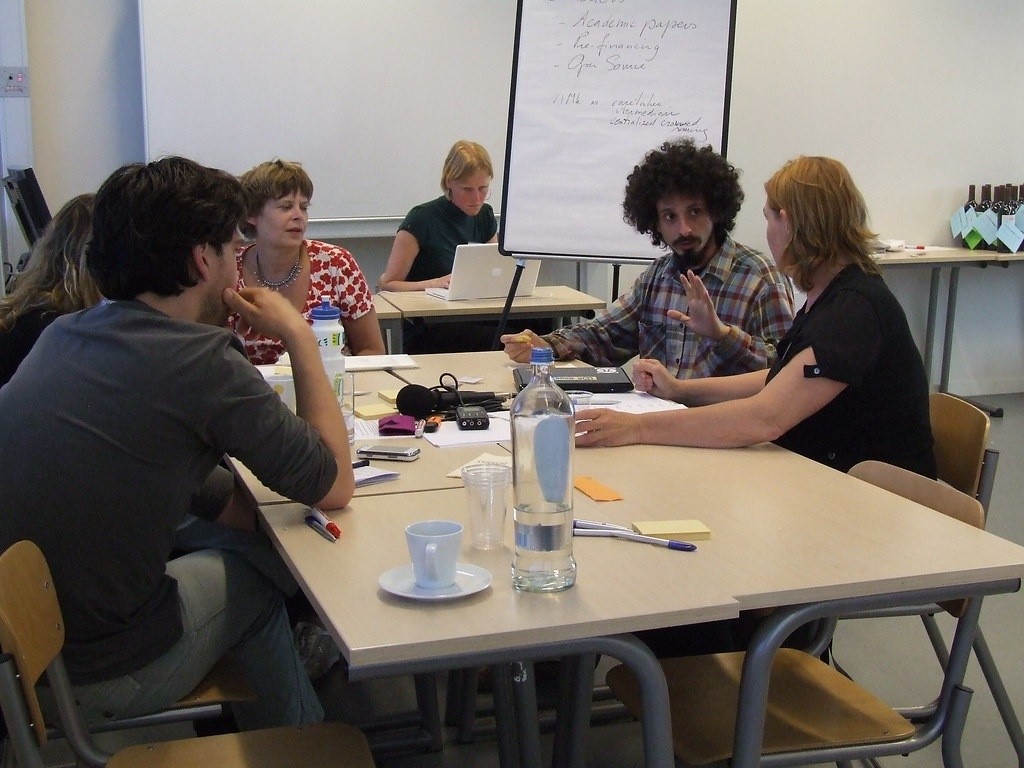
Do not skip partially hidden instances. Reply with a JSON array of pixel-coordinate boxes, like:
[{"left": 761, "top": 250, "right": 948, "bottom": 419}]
[{"left": 291, "top": 619, "right": 341, "bottom": 683}]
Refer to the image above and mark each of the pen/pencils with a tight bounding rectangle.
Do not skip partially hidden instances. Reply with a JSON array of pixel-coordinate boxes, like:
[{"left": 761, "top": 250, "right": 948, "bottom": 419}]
[
  {"left": 612, "top": 530, "right": 697, "bottom": 552},
  {"left": 342, "top": 391, "right": 374, "bottom": 396},
  {"left": 572, "top": 399, "right": 621, "bottom": 405},
  {"left": 353, "top": 460, "right": 369, "bottom": 469},
  {"left": 305, "top": 516, "right": 336, "bottom": 542},
  {"left": 573, "top": 520, "right": 640, "bottom": 537},
  {"left": 885, "top": 250, "right": 902, "bottom": 252}
]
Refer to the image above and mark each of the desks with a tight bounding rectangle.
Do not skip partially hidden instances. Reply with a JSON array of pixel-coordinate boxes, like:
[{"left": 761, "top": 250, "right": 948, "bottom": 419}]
[
  {"left": 869, "top": 245, "right": 1024, "bottom": 417},
  {"left": 221, "top": 350, "right": 1024, "bottom": 768},
  {"left": 369, "top": 285, "right": 607, "bottom": 356}
]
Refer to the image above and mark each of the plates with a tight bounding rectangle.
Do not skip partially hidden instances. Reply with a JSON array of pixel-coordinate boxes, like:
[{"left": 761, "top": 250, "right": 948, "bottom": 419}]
[{"left": 378, "top": 562, "right": 492, "bottom": 603}]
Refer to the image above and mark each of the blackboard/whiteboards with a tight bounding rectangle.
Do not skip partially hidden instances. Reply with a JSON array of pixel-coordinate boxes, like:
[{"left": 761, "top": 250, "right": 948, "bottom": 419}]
[{"left": 499, "top": 0, "right": 737, "bottom": 265}]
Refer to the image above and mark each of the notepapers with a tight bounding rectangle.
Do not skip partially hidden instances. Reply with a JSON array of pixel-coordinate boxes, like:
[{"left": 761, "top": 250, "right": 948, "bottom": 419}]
[
  {"left": 354, "top": 402, "right": 397, "bottom": 421},
  {"left": 378, "top": 389, "right": 398, "bottom": 403},
  {"left": 632, "top": 518, "right": 710, "bottom": 542}
]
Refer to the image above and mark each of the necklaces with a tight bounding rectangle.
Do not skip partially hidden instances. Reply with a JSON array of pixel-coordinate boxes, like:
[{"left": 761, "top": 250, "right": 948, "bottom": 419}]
[{"left": 252, "top": 249, "right": 303, "bottom": 290}]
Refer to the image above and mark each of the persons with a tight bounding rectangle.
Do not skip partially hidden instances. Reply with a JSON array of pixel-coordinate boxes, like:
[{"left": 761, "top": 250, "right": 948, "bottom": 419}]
[
  {"left": 0, "top": 193, "right": 104, "bottom": 388},
  {"left": 565, "top": 156, "right": 938, "bottom": 664},
  {"left": 379, "top": 139, "right": 504, "bottom": 355},
  {"left": 474, "top": 141, "right": 795, "bottom": 707},
  {"left": 221, "top": 158, "right": 386, "bottom": 365},
  {"left": 0, "top": 156, "right": 342, "bottom": 736}
]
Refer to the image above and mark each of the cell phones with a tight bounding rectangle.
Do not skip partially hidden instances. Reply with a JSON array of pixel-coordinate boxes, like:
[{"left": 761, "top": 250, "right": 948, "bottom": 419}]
[{"left": 355, "top": 444, "right": 421, "bottom": 461}]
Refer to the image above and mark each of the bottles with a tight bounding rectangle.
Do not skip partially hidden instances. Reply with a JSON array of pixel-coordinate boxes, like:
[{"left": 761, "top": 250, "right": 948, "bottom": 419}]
[
  {"left": 311, "top": 296, "right": 347, "bottom": 383},
  {"left": 509, "top": 347, "right": 576, "bottom": 594},
  {"left": 992, "top": 184, "right": 1024, "bottom": 253},
  {"left": 975, "top": 185, "right": 992, "bottom": 250},
  {"left": 962, "top": 185, "right": 978, "bottom": 248}
]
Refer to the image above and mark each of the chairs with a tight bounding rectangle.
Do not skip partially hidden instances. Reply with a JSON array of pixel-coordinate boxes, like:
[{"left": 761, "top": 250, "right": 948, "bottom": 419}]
[
  {"left": 605, "top": 459, "right": 984, "bottom": 768},
  {"left": 0, "top": 540, "right": 377, "bottom": 768},
  {"left": 806, "top": 392, "right": 1023, "bottom": 768}
]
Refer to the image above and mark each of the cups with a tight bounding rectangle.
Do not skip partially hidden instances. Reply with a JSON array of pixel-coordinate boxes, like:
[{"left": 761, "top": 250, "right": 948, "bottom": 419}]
[
  {"left": 328, "top": 372, "right": 355, "bottom": 447},
  {"left": 564, "top": 390, "right": 593, "bottom": 438},
  {"left": 461, "top": 463, "right": 512, "bottom": 550},
  {"left": 266, "top": 376, "right": 293, "bottom": 409},
  {"left": 404, "top": 521, "right": 464, "bottom": 590}
]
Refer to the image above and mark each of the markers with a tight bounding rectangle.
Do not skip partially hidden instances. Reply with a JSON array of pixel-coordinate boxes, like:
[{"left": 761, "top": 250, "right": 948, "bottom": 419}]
[
  {"left": 310, "top": 507, "right": 340, "bottom": 537},
  {"left": 905, "top": 246, "right": 925, "bottom": 249}
]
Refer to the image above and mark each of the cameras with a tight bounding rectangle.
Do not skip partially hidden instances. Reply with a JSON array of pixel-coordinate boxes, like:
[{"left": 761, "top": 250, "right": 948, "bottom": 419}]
[{"left": 456, "top": 406, "right": 490, "bottom": 431}]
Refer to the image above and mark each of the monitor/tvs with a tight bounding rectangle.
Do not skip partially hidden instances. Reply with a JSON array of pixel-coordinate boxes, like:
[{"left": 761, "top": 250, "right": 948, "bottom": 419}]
[{"left": 3, "top": 165, "right": 53, "bottom": 249}]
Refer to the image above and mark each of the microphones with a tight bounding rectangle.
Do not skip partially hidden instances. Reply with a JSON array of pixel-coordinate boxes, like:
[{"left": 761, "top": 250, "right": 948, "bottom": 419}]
[{"left": 395, "top": 385, "right": 520, "bottom": 420}]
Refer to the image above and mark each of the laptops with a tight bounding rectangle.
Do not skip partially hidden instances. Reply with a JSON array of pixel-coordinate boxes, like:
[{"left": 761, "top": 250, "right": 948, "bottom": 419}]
[
  {"left": 425, "top": 244, "right": 542, "bottom": 300},
  {"left": 512, "top": 367, "right": 635, "bottom": 393}
]
